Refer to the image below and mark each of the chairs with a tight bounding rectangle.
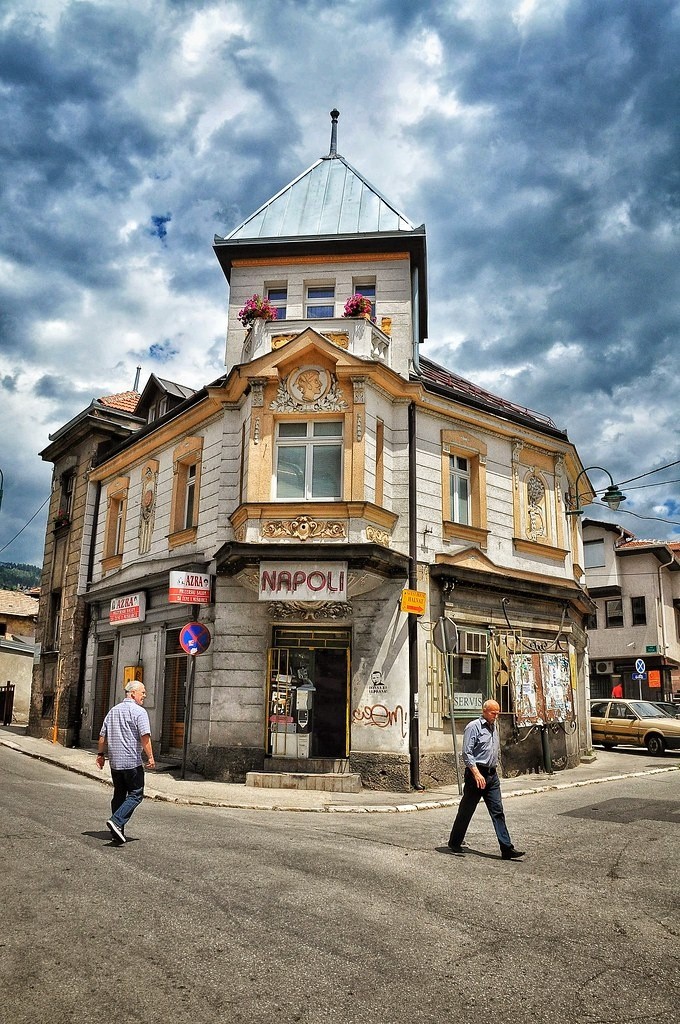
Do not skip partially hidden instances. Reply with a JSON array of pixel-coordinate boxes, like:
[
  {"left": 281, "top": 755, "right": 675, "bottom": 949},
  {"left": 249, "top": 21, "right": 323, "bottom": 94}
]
[{"left": 611, "top": 706, "right": 633, "bottom": 718}]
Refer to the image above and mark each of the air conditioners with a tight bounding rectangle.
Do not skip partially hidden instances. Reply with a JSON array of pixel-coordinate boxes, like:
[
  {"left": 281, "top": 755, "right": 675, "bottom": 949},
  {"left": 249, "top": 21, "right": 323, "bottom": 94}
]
[{"left": 596, "top": 661, "right": 614, "bottom": 674}]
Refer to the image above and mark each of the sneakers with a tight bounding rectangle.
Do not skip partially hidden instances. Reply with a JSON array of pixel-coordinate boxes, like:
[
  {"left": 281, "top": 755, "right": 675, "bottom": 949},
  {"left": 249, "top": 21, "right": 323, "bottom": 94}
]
[{"left": 106, "top": 819, "right": 126, "bottom": 843}]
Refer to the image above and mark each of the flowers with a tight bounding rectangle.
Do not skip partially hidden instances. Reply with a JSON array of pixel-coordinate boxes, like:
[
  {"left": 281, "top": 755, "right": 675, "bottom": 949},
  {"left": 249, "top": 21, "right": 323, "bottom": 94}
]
[
  {"left": 237, "top": 293, "right": 278, "bottom": 332},
  {"left": 341, "top": 294, "right": 372, "bottom": 318},
  {"left": 53, "top": 509, "right": 70, "bottom": 522}
]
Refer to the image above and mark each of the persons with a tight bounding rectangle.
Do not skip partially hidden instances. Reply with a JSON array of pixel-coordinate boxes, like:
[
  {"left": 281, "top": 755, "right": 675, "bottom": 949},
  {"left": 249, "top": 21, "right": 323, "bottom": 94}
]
[
  {"left": 94, "top": 680, "right": 156, "bottom": 845},
  {"left": 446, "top": 698, "right": 527, "bottom": 861}
]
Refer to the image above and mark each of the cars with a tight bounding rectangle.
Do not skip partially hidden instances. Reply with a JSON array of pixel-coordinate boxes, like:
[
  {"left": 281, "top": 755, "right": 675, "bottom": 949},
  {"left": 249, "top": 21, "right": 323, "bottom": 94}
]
[
  {"left": 590, "top": 699, "right": 680, "bottom": 757},
  {"left": 650, "top": 700, "right": 680, "bottom": 720}
]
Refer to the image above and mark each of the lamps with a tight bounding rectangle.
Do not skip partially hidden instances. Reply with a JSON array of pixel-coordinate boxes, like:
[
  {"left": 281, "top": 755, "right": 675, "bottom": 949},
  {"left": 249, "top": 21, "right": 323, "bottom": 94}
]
[{"left": 566, "top": 467, "right": 626, "bottom": 516}]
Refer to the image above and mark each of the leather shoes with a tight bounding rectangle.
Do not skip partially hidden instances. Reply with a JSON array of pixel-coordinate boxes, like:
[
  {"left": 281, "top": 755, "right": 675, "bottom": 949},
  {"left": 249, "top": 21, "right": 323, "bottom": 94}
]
[
  {"left": 448, "top": 840, "right": 466, "bottom": 853},
  {"left": 501, "top": 847, "right": 526, "bottom": 860}
]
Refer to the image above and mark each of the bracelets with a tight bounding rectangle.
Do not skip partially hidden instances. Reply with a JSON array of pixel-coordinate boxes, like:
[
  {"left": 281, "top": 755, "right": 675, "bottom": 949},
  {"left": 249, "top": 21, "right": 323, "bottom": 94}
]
[{"left": 98, "top": 752, "right": 104, "bottom": 756}]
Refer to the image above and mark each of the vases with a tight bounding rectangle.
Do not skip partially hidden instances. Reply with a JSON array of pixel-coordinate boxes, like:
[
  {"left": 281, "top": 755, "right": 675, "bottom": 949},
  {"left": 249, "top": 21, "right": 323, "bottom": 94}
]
[
  {"left": 357, "top": 312, "right": 371, "bottom": 320},
  {"left": 55, "top": 519, "right": 68, "bottom": 529},
  {"left": 253, "top": 309, "right": 269, "bottom": 318}
]
